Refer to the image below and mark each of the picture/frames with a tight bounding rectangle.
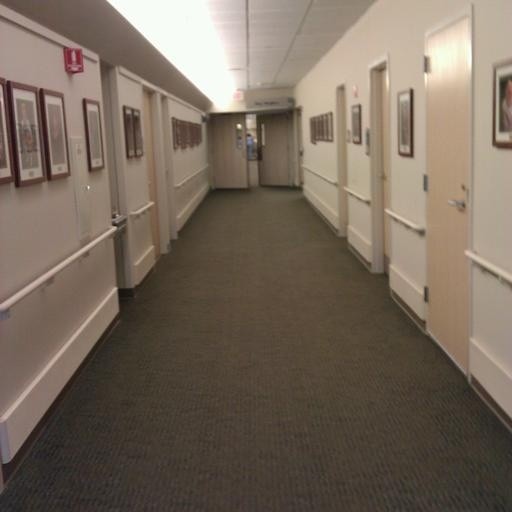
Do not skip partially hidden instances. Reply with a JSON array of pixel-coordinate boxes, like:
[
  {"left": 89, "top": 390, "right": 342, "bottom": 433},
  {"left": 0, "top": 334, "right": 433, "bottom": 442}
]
[
  {"left": 492, "top": 58, "right": 512, "bottom": 149},
  {"left": 123, "top": 105, "right": 144, "bottom": 159},
  {"left": 350, "top": 104, "right": 362, "bottom": 144},
  {"left": 0, "top": 77, "right": 71, "bottom": 188},
  {"left": 172, "top": 116, "right": 202, "bottom": 150},
  {"left": 82, "top": 98, "right": 104, "bottom": 173},
  {"left": 397, "top": 88, "right": 413, "bottom": 157},
  {"left": 309, "top": 112, "right": 333, "bottom": 145}
]
